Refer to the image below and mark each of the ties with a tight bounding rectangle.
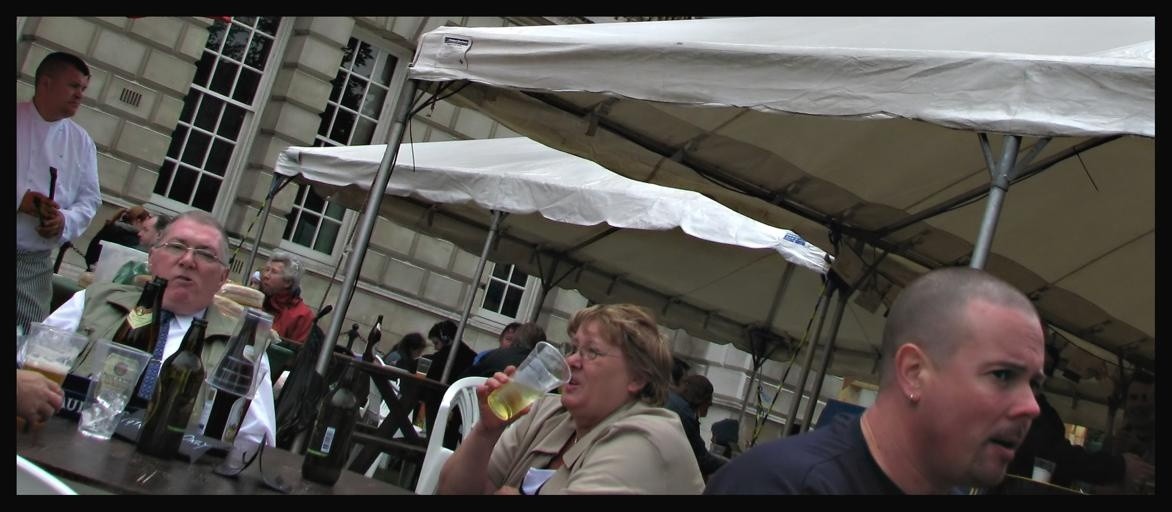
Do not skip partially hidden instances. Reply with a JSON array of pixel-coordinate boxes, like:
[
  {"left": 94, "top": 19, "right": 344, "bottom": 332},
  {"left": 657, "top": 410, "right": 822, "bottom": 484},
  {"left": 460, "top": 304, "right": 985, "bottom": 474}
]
[{"left": 137, "top": 310, "right": 175, "bottom": 402}]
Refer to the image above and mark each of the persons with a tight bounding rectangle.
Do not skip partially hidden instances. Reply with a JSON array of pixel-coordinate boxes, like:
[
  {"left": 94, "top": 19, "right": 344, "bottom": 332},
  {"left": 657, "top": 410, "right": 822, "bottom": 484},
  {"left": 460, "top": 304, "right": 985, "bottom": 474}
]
[
  {"left": 701, "top": 266, "right": 1046, "bottom": 495},
  {"left": 1007, "top": 372, "right": 1155, "bottom": 496},
  {"left": 17, "top": 206, "right": 315, "bottom": 449},
  {"left": 664, "top": 374, "right": 715, "bottom": 486},
  {"left": 16, "top": 52, "right": 102, "bottom": 336},
  {"left": 433, "top": 306, "right": 707, "bottom": 496},
  {"left": 382, "top": 321, "right": 478, "bottom": 429},
  {"left": 451, "top": 321, "right": 559, "bottom": 440}
]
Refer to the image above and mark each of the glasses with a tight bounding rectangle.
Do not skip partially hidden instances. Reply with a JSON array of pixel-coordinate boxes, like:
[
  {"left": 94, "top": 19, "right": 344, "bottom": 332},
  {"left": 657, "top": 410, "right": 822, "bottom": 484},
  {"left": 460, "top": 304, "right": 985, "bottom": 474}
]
[
  {"left": 558, "top": 341, "right": 627, "bottom": 363},
  {"left": 154, "top": 242, "right": 229, "bottom": 269}
]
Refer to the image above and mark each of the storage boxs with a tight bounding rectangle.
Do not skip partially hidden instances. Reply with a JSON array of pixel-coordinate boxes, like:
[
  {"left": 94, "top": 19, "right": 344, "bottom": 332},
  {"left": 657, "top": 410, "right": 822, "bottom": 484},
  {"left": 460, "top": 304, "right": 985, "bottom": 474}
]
[{"left": 93, "top": 239, "right": 151, "bottom": 289}]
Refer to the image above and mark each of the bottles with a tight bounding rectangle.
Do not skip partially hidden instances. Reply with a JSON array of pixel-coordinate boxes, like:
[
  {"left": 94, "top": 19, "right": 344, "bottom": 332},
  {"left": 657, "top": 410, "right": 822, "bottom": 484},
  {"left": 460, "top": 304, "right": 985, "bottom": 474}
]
[
  {"left": 358, "top": 312, "right": 389, "bottom": 363},
  {"left": 196, "top": 308, "right": 262, "bottom": 459},
  {"left": 102, "top": 276, "right": 171, "bottom": 419},
  {"left": 297, "top": 352, "right": 366, "bottom": 489},
  {"left": 139, "top": 318, "right": 213, "bottom": 454}
]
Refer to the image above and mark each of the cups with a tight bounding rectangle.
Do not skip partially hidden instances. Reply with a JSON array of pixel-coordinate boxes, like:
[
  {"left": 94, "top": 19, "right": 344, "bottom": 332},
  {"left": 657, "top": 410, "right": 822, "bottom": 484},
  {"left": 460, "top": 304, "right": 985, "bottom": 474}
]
[
  {"left": 485, "top": 341, "right": 573, "bottom": 422},
  {"left": 70, "top": 338, "right": 152, "bottom": 440},
  {"left": 201, "top": 309, "right": 281, "bottom": 402},
  {"left": 710, "top": 443, "right": 742, "bottom": 460},
  {"left": 415, "top": 357, "right": 432, "bottom": 380},
  {"left": 21, "top": 317, "right": 82, "bottom": 433},
  {"left": 1030, "top": 456, "right": 1063, "bottom": 482}
]
